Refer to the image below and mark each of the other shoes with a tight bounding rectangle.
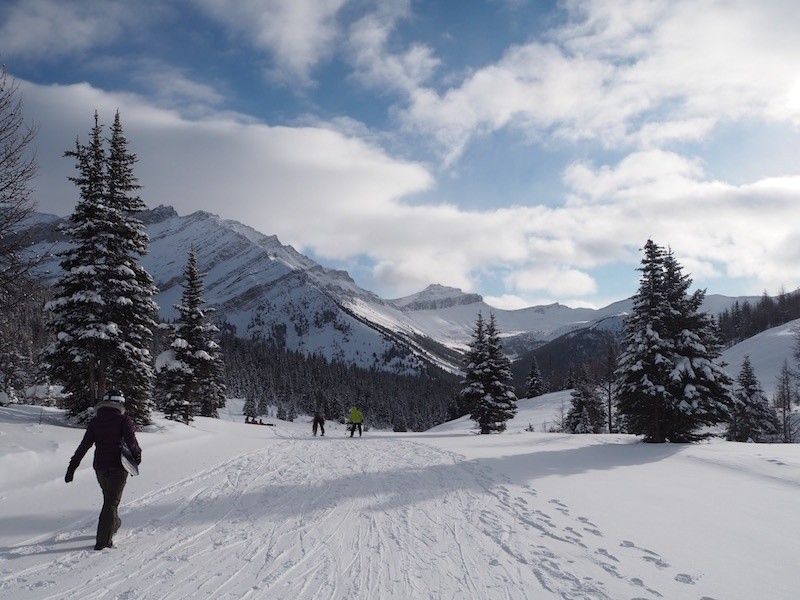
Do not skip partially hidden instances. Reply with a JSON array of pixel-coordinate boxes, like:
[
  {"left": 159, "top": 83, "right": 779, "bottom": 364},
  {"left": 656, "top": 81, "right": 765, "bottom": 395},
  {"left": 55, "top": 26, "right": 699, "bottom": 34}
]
[
  {"left": 94, "top": 540, "right": 117, "bottom": 550},
  {"left": 113, "top": 519, "right": 121, "bottom": 533}
]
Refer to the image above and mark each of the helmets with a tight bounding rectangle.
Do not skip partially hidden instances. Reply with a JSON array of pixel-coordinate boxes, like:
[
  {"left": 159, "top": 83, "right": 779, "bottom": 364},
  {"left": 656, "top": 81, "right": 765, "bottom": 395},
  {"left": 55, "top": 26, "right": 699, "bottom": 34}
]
[{"left": 104, "top": 389, "right": 125, "bottom": 405}]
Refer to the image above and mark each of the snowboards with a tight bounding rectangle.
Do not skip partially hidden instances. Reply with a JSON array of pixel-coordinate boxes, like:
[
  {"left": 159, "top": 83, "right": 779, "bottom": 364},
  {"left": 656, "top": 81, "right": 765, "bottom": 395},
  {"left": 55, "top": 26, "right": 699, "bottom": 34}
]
[{"left": 121, "top": 442, "right": 138, "bottom": 476}]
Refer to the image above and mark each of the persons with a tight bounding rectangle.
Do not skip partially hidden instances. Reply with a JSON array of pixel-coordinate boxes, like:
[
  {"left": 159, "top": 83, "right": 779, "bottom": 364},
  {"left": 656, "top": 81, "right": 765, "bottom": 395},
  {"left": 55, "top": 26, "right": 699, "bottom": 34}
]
[
  {"left": 348, "top": 406, "right": 364, "bottom": 438},
  {"left": 312, "top": 400, "right": 325, "bottom": 437},
  {"left": 64, "top": 389, "right": 142, "bottom": 551}
]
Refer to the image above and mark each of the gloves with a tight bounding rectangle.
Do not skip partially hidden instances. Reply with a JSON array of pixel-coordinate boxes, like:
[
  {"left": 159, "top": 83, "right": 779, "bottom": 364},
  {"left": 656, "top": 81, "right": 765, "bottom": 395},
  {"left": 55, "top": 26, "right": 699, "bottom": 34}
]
[{"left": 65, "top": 465, "right": 77, "bottom": 482}]
